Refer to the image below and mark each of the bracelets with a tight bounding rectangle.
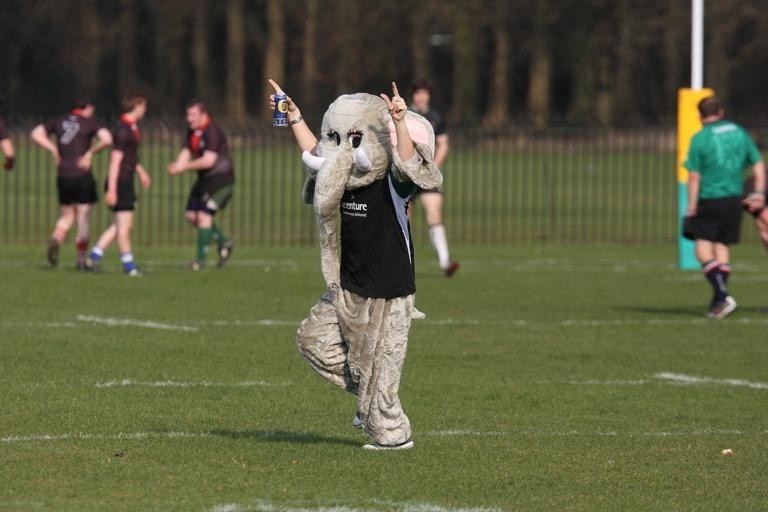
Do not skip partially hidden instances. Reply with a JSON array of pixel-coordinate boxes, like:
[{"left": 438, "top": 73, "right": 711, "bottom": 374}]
[{"left": 750, "top": 188, "right": 764, "bottom": 195}]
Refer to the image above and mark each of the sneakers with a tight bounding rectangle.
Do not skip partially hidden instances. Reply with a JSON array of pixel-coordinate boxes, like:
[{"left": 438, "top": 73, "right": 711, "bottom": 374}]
[
  {"left": 47, "top": 238, "right": 234, "bottom": 276},
  {"left": 444, "top": 263, "right": 458, "bottom": 277},
  {"left": 352, "top": 414, "right": 362, "bottom": 430},
  {"left": 362, "top": 440, "right": 414, "bottom": 450},
  {"left": 707, "top": 296, "right": 736, "bottom": 319}
]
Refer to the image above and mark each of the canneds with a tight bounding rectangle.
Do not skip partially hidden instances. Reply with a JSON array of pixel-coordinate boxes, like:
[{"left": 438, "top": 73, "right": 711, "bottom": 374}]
[{"left": 272, "top": 95, "right": 289, "bottom": 129}]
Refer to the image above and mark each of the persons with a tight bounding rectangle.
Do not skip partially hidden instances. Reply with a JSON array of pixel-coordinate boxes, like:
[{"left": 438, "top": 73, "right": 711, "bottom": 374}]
[
  {"left": 31, "top": 92, "right": 112, "bottom": 266},
  {"left": 1, "top": 117, "right": 15, "bottom": 171},
  {"left": 743, "top": 170, "right": 768, "bottom": 245},
  {"left": 682, "top": 97, "right": 765, "bottom": 320},
  {"left": 84, "top": 91, "right": 150, "bottom": 276},
  {"left": 265, "top": 78, "right": 443, "bottom": 452},
  {"left": 168, "top": 100, "right": 235, "bottom": 270},
  {"left": 403, "top": 85, "right": 459, "bottom": 276}
]
